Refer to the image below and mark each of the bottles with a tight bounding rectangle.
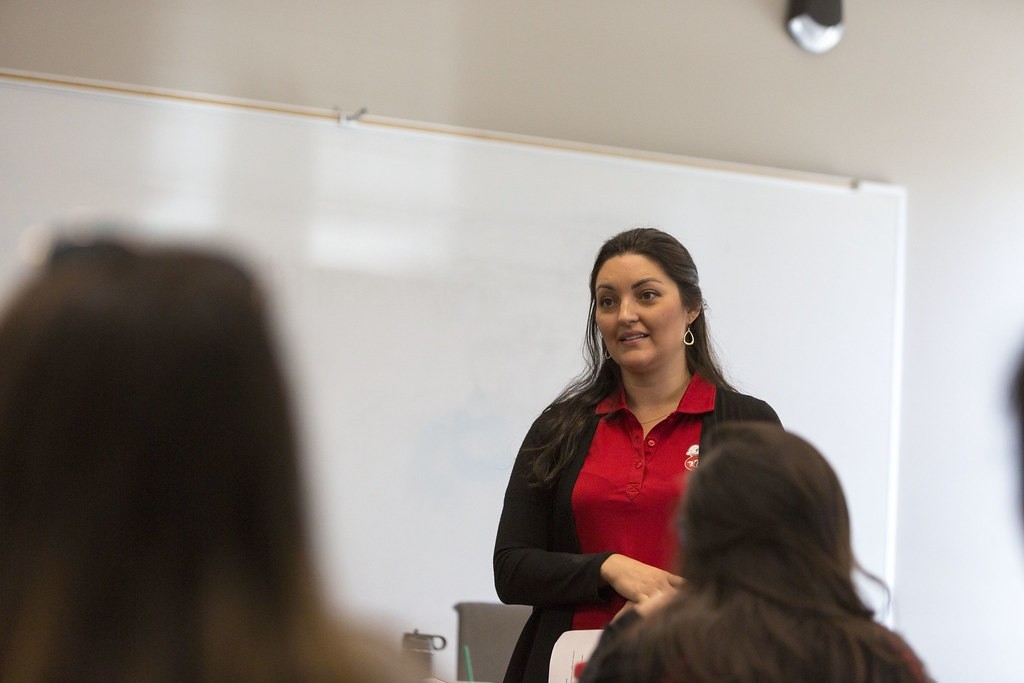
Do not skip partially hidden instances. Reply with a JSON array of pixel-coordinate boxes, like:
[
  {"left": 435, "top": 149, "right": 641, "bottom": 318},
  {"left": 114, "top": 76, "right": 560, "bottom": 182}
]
[{"left": 401, "top": 628, "right": 446, "bottom": 679}]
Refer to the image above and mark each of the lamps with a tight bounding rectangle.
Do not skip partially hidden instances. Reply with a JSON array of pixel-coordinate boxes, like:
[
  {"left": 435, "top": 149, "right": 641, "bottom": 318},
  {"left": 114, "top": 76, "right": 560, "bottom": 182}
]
[{"left": 787, "top": 0, "right": 844, "bottom": 53}]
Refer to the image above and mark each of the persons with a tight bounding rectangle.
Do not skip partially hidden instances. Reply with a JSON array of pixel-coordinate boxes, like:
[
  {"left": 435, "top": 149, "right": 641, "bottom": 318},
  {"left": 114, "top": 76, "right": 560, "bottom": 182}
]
[
  {"left": 494, "top": 227, "right": 785, "bottom": 683},
  {"left": 678, "top": 425, "right": 871, "bottom": 616},
  {"left": 0, "top": 243, "right": 417, "bottom": 683},
  {"left": 580, "top": 590, "right": 938, "bottom": 683}
]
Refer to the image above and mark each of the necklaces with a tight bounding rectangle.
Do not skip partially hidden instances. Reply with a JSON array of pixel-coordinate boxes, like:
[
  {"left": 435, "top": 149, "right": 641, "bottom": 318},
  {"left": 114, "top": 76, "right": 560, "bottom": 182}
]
[{"left": 641, "top": 413, "right": 670, "bottom": 424}]
